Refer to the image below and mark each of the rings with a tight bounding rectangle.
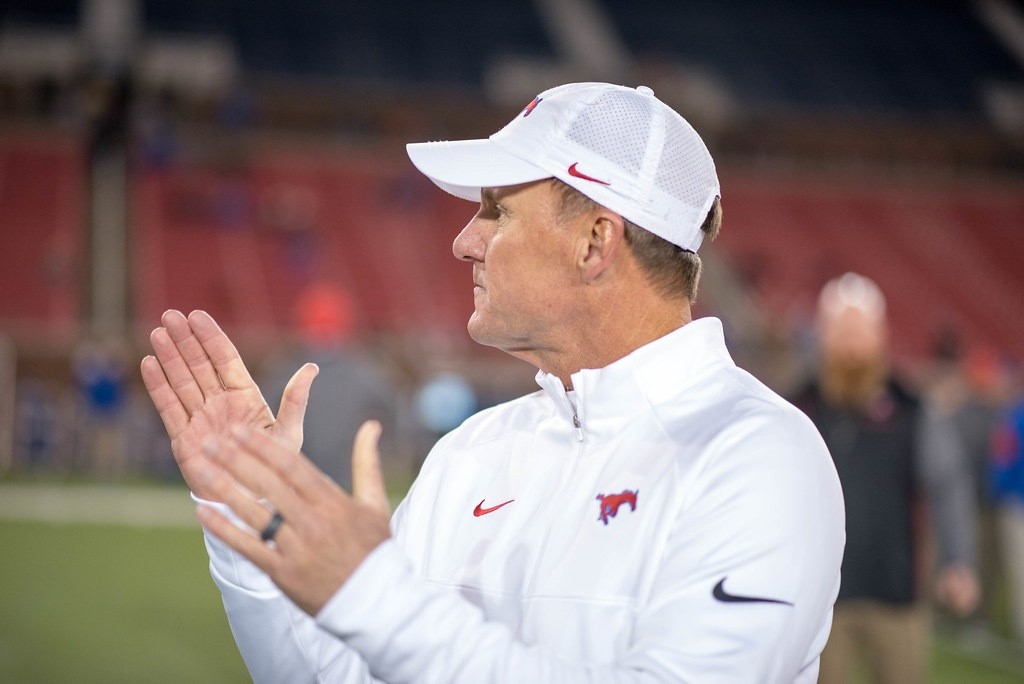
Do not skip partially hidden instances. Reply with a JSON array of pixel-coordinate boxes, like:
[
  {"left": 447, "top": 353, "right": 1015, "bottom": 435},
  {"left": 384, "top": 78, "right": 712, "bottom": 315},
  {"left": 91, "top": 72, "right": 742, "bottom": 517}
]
[{"left": 262, "top": 514, "right": 284, "bottom": 541}]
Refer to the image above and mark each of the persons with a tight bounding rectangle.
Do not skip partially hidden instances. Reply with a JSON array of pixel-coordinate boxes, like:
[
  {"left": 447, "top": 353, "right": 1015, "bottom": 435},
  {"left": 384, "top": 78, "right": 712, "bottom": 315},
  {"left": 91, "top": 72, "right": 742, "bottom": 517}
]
[
  {"left": 141, "top": 83, "right": 845, "bottom": 684},
  {"left": 787, "top": 275, "right": 1024, "bottom": 684},
  {"left": 65, "top": 350, "right": 134, "bottom": 483},
  {"left": 411, "top": 335, "right": 472, "bottom": 481}
]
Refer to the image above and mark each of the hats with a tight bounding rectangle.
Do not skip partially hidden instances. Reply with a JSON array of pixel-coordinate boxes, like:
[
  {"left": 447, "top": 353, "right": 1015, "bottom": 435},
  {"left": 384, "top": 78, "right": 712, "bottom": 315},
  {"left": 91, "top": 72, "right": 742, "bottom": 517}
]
[{"left": 404, "top": 79, "right": 725, "bottom": 255}]
